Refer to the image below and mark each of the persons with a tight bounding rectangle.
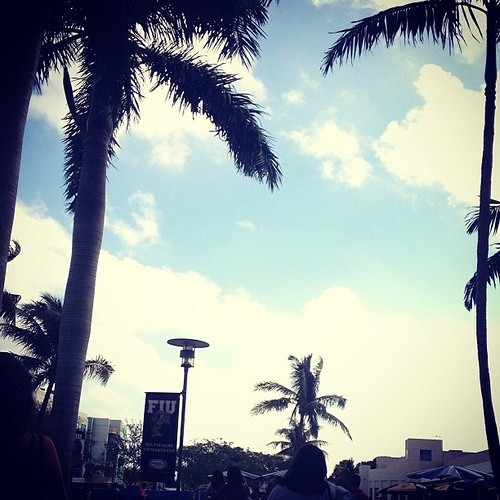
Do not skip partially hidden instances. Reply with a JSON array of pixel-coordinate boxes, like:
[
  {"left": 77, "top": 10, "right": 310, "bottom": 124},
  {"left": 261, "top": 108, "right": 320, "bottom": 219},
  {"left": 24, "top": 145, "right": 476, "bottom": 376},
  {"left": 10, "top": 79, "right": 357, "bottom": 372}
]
[
  {"left": 348, "top": 474, "right": 369, "bottom": 500},
  {"left": 138, "top": 483, "right": 148, "bottom": 500},
  {"left": 216, "top": 466, "right": 250, "bottom": 500},
  {"left": 112, "top": 482, "right": 120, "bottom": 500},
  {"left": 267, "top": 443, "right": 352, "bottom": 500},
  {"left": 205, "top": 469, "right": 226, "bottom": 500},
  {"left": 0, "top": 353, "right": 66, "bottom": 500}
]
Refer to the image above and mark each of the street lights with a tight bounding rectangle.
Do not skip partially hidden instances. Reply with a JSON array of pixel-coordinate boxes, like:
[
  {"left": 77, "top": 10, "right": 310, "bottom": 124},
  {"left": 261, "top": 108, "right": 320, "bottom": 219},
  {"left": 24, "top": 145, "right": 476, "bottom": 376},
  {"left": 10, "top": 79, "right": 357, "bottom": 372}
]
[{"left": 167, "top": 339, "right": 208, "bottom": 491}]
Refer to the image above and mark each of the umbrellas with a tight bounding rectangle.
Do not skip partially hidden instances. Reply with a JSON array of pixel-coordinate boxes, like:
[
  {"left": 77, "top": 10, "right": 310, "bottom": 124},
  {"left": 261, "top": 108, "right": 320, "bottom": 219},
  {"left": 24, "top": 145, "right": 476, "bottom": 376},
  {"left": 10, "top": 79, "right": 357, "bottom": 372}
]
[
  {"left": 405, "top": 464, "right": 495, "bottom": 493},
  {"left": 378, "top": 481, "right": 431, "bottom": 493}
]
